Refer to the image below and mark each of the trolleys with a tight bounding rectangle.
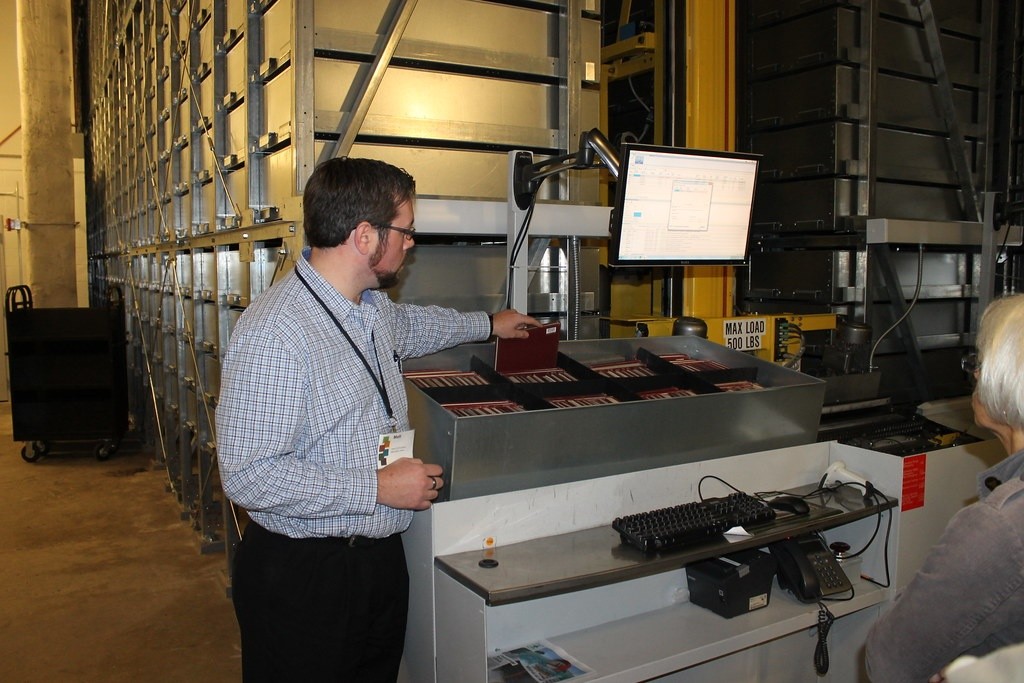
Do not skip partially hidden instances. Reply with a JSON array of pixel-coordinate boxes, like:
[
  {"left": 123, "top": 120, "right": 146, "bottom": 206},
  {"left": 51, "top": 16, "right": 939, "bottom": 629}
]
[{"left": 4, "top": 285, "right": 129, "bottom": 463}]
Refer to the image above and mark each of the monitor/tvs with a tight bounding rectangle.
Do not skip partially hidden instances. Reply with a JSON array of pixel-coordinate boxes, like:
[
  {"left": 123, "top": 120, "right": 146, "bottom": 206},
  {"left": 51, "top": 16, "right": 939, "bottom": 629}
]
[{"left": 608, "top": 142, "right": 765, "bottom": 267}]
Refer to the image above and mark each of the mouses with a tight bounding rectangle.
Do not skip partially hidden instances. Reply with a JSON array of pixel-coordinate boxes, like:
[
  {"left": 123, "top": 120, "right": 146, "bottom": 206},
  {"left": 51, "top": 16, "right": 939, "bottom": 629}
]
[{"left": 768, "top": 496, "right": 810, "bottom": 515}]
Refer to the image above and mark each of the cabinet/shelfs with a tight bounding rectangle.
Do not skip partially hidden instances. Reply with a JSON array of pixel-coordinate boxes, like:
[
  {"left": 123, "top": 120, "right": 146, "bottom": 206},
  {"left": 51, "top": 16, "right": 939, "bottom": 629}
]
[
  {"left": 432, "top": 481, "right": 898, "bottom": 683},
  {"left": 5, "top": 283, "right": 128, "bottom": 460}
]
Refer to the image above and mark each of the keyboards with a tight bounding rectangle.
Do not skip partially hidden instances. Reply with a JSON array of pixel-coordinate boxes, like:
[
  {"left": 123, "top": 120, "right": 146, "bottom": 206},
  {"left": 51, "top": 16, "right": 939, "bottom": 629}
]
[{"left": 611, "top": 492, "right": 777, "bottom": 551}]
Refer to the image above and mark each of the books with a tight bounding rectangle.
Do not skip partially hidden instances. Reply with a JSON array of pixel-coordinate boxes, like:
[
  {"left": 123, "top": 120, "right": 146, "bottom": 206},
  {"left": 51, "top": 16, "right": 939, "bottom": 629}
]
[
  {"left": 495, "top": 320, "right": 580, "bottom": 382},
  {"left": 440, "top": 401, "right": 527, "bottom": 417},
  {"left": 542, "top": 393, "right": 622, "bottom": 408},
  {"left": 714, "top": 381, "right": 764, "bottom": 392},
  {"left": 638, "top": 386, "right": 699, "bottom": 401},
  {"left": 403, "top": 368, "right": 491, "bottom": 388},
  {"left": 586, "top": 360, "right": 657, "bottom": 378},
  {"left": 658, "top": 354, "right": 730, "bottom": 372}
]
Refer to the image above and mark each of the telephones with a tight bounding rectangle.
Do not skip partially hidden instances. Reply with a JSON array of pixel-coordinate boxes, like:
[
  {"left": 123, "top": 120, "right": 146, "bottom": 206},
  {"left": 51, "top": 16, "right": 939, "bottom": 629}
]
[{"left": 768, "top": 534, "right": 852, "bottom": 603}]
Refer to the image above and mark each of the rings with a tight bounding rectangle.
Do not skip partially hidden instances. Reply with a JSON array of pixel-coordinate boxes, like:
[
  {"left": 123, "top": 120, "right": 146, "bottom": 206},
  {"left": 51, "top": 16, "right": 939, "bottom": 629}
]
[{"left": 429, "top": 476, "right": 436, "bottom": 489}]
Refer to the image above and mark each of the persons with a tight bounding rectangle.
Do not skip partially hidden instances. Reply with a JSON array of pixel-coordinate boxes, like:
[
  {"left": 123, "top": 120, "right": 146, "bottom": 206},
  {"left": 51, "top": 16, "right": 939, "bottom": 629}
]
[
  {"left": 215, "top": 157, "right": 544, "bottom": 683},
  {"left": 865, "top": 296, "right": 1024, "bottom": 683}
]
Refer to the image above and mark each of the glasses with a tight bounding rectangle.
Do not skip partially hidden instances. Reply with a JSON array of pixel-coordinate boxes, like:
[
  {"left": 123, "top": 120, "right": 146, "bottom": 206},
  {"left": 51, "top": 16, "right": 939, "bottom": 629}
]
[
  {"left": 961, "top": 352, "right": 984, "bottom": 373},
  {"left": 367, "top": 220, "right": 416, "bottom": 240}
]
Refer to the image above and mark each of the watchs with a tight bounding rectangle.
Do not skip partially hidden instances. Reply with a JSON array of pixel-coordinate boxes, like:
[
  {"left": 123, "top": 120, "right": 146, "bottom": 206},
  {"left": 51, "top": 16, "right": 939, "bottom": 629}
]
[{"left": 487, "top": 312, "right": 493, "bottom": 335}]
